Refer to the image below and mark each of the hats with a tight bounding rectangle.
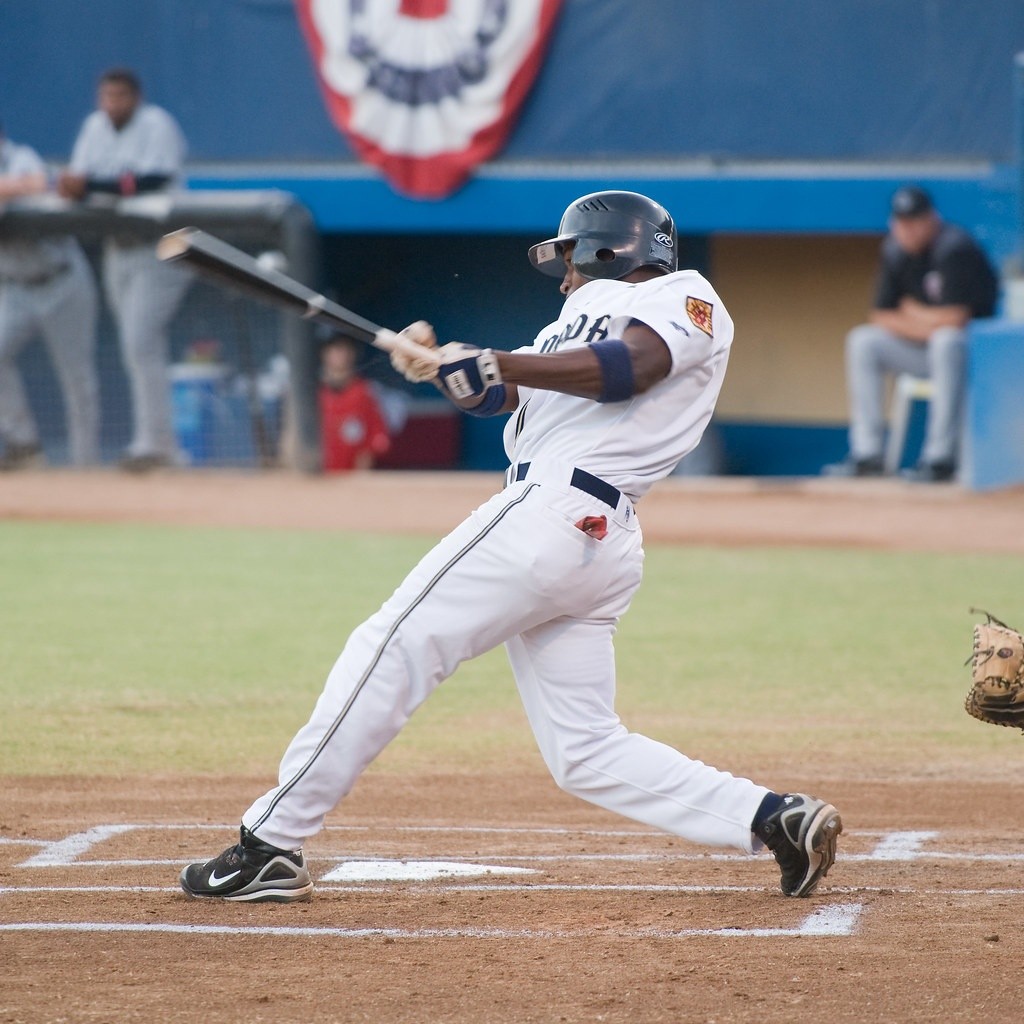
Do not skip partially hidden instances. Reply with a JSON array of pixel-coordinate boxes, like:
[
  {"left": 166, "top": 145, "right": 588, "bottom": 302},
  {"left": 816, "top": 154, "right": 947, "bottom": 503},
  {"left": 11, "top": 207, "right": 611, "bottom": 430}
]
[{"left": 889, "top": 185, "right": 931, "bottom": 217}]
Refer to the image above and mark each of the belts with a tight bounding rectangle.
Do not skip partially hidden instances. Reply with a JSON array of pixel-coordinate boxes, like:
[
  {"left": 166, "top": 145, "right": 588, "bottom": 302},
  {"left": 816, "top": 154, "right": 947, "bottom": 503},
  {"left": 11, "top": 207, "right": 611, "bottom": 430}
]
[{"left": 503, "top": 462, "right": 620, "bottom": 510}]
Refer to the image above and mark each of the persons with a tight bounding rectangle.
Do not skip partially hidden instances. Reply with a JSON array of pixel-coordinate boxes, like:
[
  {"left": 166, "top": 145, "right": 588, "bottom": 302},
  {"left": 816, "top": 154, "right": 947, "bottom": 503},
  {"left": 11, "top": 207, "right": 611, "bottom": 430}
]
[
  {"left": 0, "top": 65, "right": 393, "bottom": 472},
  {"left": 821, "top": 185, "right": 1001, "bottom": 482},
  {"left": 179, "top": 189, "right": 843, "bottom": 905}
]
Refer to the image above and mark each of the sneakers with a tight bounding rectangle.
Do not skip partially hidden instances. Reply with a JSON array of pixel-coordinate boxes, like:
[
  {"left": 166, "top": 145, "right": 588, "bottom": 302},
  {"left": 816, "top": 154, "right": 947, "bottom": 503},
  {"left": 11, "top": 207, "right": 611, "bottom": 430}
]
[
  {"left": 178, "top": 825, "right": 313, "bottom": 902},
  {"left": 756, "top": 791, "right": 843, "bottom": 898}
]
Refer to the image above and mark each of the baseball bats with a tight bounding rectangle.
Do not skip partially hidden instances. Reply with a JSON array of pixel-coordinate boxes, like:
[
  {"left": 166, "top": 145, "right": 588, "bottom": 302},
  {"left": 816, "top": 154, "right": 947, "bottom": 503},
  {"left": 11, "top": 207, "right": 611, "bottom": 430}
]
[{"left": 154, "top": 232, "right": 445, "bottom": 373}]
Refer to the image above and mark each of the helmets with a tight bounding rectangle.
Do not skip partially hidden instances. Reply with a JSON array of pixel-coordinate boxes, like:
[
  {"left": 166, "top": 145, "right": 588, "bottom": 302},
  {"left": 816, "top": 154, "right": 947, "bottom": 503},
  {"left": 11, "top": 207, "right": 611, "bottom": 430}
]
[{"left": 528, "top": 190, "right": 678, "bottom": 282}]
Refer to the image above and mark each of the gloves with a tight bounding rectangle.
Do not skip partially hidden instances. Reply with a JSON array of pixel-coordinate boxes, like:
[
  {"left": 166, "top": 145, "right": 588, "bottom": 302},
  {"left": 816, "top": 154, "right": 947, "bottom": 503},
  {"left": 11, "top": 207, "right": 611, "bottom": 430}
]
[
  {"left": 439, "top": 339, "right": 504, "bottom": 400},
  {"left": 389, "top": 321, "right": 440, "bottom": 384}
]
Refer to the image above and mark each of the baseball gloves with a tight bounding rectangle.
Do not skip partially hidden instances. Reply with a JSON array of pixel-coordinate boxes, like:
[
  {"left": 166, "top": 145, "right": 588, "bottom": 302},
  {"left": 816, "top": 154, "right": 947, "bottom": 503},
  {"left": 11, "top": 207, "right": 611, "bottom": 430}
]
[{"left": 964, "top": 607, "right": 1024, "bottom": 728}]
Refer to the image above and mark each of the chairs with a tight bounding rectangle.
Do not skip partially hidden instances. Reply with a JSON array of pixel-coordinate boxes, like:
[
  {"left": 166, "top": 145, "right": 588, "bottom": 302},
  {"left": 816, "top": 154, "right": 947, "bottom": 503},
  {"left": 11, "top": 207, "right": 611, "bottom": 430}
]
[{"left": 883, "top": 279, "right": 1024, "bottom": 476}]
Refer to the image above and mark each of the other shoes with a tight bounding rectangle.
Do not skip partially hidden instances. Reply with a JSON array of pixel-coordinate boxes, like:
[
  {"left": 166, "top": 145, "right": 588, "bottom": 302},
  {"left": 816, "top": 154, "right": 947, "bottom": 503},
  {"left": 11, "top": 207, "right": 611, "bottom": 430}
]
[
  {"left": 904, "top": 461, "right": 953, "bottom": 480},
  {"left": 820, "top": 454, "right": 885, "bottom": 477}
]
